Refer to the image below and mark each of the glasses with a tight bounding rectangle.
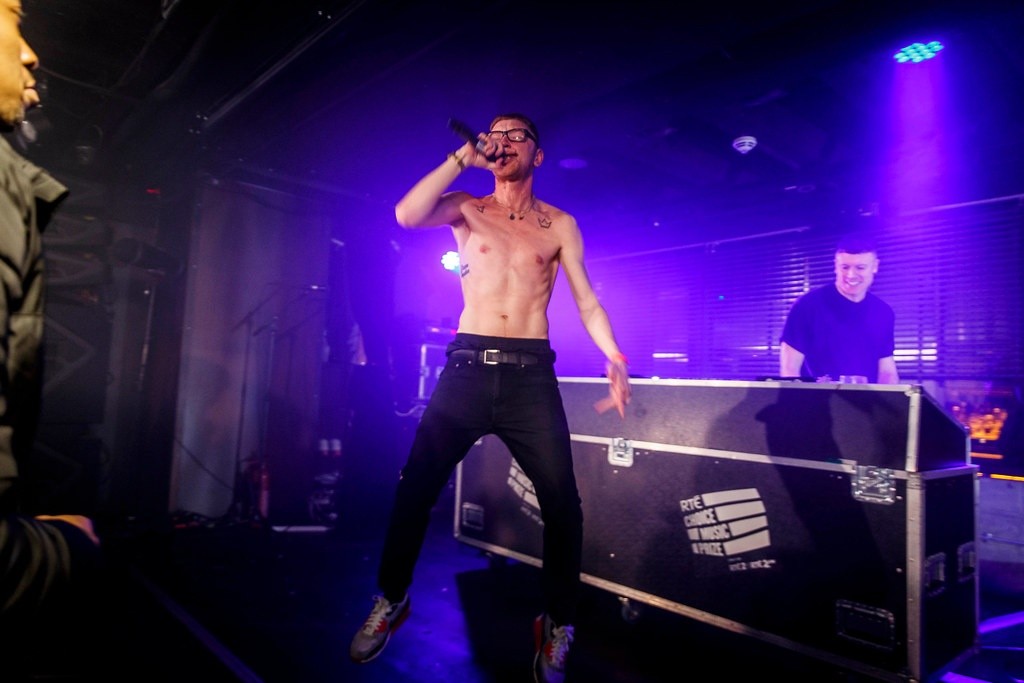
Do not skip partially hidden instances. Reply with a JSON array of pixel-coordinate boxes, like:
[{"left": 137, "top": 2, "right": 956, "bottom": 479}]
[{"left": 489, "top": 128, "right": 538, "bottom": 143}]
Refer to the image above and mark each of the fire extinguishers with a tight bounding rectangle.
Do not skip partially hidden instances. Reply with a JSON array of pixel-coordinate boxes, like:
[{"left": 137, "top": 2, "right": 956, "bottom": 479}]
[{"left": 242, "top": 456, "right": 270, "bottom": 530}]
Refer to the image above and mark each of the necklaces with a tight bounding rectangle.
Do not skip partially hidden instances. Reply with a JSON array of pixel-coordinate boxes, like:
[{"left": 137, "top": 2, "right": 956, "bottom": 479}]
[{"left": 492, "top": 194, "right": 538, "bottom": 219}]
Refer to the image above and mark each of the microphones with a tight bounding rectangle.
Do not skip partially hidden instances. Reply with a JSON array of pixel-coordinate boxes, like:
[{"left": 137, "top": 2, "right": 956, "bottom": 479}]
[{"left": 448, "top": 119, "right": 507, "bottom": 162}]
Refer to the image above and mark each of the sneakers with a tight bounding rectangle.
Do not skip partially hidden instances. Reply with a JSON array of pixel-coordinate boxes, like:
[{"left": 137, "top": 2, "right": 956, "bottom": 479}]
[
  {"left": 533, "top": 612, "right": 576, "bottom": 683},
  {"left": 350, "top": 593, "right": 411, "bottom": 664}
]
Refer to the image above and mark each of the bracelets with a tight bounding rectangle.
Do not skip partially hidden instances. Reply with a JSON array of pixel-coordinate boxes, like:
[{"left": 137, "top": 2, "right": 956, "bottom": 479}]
[
  {"left": 449, "top": 153, "right": 468, "bottom": 173},
  {"left": 612, "top": 354, "right": 630, "bottom": 367}
]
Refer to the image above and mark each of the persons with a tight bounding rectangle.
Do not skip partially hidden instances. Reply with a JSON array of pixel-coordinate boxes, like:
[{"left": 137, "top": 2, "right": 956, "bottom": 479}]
[
  {"left": 0, "top": 0, "right": 100, "bottom": 683},
  {"left": 350, "top": 112, "right": 630, "bottom": 683},
  {"left": 780, "top": 233, "right": 899, "bottom": 387}
]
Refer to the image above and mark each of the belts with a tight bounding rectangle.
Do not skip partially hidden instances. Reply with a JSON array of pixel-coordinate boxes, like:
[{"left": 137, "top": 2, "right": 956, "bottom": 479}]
[{"left": 449, "top": 346, "right": 539, "bottom": 369}]
